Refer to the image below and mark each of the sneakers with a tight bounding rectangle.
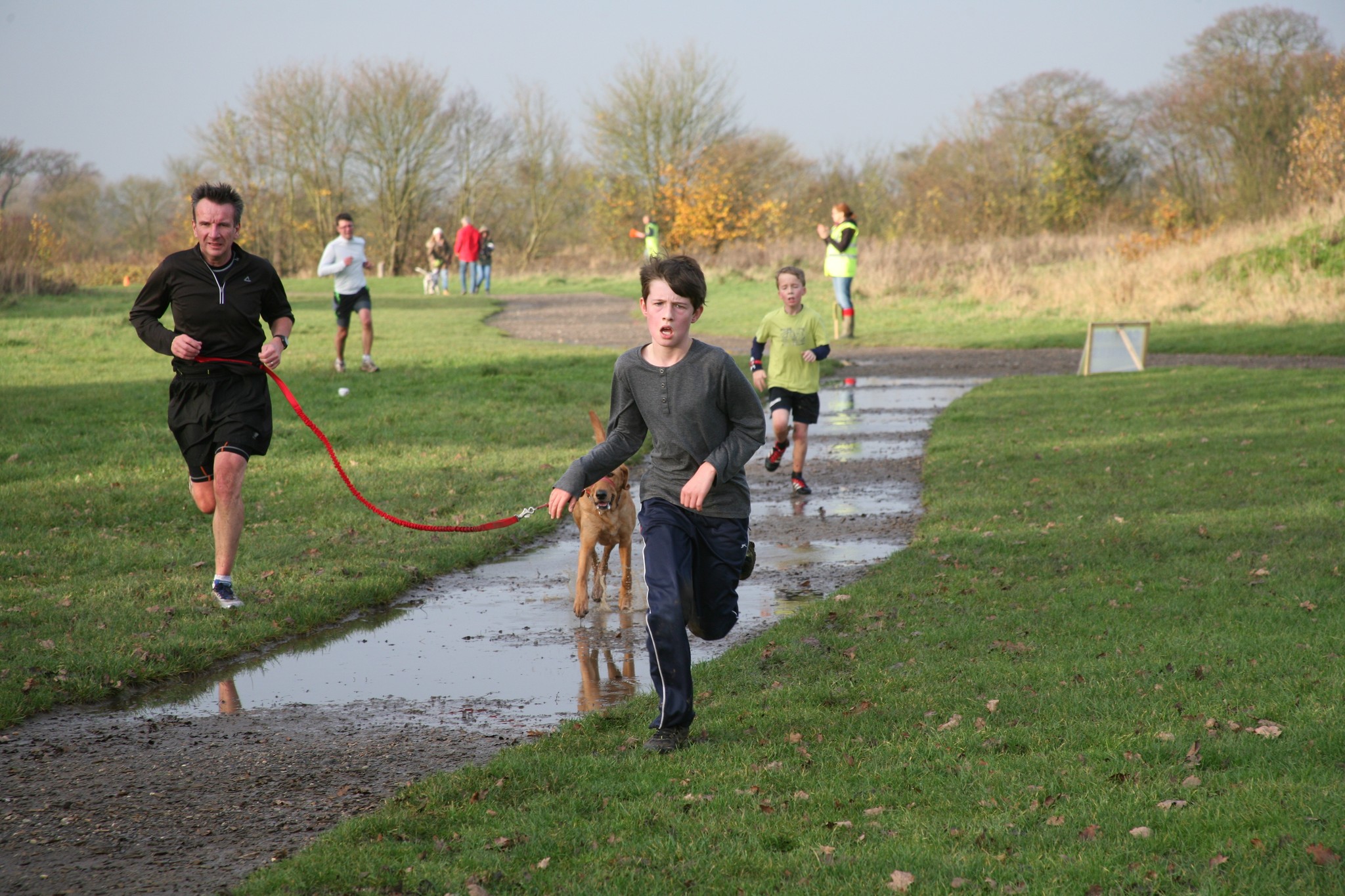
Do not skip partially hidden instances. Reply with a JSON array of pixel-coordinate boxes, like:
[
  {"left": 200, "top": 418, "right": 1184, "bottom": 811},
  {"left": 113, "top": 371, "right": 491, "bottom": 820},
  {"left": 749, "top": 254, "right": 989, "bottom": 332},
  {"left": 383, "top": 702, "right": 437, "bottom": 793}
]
[
  {"left": 641, "top": 726, "right": 689, "bottom": 754},
  {"left": 211, "top": 578, "right": 245, "bottom": 609},
  {"left": 765, "top": 440, "right": 789, "bottom": 471},
  {"left": 336, "top": 362, "right": 345, "bottom": 373},
  {"left": 738, "top": 541, "right": 756, "bottom": 580},
  {"left": 792, "top": 476, "right": 811, "bottom": 494},
  {"left": 361, "top": 360, "right": 379, "bottom": 372}
]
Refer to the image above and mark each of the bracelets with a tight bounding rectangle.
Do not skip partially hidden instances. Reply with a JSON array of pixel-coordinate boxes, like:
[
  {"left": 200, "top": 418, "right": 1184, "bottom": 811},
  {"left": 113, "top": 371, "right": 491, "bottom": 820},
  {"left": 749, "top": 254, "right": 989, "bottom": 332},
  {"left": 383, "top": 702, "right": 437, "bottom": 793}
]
[{"left": 749, "top": 360, "right": 762, "bottom": 367}]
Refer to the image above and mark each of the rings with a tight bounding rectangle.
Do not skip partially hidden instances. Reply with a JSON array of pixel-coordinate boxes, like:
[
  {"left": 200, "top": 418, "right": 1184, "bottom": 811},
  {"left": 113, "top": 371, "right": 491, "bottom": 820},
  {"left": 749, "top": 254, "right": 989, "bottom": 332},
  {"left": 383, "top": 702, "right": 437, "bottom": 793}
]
[{"left": 270, "top": 361, "right": 275, "bottom": 366}]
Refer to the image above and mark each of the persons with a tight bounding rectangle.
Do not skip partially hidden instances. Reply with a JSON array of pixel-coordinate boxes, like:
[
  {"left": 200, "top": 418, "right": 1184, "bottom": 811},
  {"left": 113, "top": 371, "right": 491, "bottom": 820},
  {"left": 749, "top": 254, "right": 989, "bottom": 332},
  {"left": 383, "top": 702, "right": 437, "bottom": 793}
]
[
  {"left": 636, "top": 214, "right": 660, "bottom": 265},
  {"left": 128, "top": 182, "right": 295, "bottom": 612},
  {"left": 452, "top": 214, "right": 481, "bottom": 295},
  {"left": 474, "top": 224, "right": 495, "bottom": 295},
  {"left": 424, "top": 226, "right": 451, "bottom": 297},
  {"left": 816, "top": 201, "right": 860, "bottom": 340},
  {"left": 547, "top": 252, "right": 766, "bottom": 758},
  {"left": 317, "top": 213, "right": 381, "bottom": 374},
  {"left": 748, "top": 266, "right": 831, "bottom": 496}
]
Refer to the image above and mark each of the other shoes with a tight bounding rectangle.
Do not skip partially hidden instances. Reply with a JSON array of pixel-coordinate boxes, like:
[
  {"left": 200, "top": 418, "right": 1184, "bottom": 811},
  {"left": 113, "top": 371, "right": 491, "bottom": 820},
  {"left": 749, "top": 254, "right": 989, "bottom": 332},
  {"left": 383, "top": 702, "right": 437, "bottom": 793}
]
[{"left": 423, "top": 288, "right": 491, "bottom": 297}]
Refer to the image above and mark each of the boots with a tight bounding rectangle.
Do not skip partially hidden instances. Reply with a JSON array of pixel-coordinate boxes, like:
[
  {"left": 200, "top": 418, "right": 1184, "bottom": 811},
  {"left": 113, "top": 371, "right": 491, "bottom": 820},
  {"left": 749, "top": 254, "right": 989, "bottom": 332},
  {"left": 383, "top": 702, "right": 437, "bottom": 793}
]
[{"left": 839, "top": 308, "right": 855, "bottom": 338}]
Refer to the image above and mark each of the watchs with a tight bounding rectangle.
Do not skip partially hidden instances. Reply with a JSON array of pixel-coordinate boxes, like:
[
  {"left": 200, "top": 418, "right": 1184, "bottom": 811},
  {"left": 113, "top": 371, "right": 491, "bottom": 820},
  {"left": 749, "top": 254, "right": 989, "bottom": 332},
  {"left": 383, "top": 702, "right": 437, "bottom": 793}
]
[{"left": 273, "top": 335, "right": 288, "bottom": 350}]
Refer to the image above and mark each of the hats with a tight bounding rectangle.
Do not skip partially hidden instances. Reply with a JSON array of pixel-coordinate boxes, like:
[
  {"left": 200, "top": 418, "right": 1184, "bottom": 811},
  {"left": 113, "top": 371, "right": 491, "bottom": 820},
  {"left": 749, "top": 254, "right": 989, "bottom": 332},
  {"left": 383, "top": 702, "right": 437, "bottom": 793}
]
[{"left": 432, "top": 227, "right": 444, "bottom": 235}]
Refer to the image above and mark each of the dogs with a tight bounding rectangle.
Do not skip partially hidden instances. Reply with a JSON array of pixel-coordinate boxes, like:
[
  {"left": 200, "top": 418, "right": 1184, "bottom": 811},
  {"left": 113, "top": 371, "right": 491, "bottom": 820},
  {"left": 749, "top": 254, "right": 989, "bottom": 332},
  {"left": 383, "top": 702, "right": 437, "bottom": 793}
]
[{"left": 573, "top": 411, "right": 636, "bottom": 618}]
